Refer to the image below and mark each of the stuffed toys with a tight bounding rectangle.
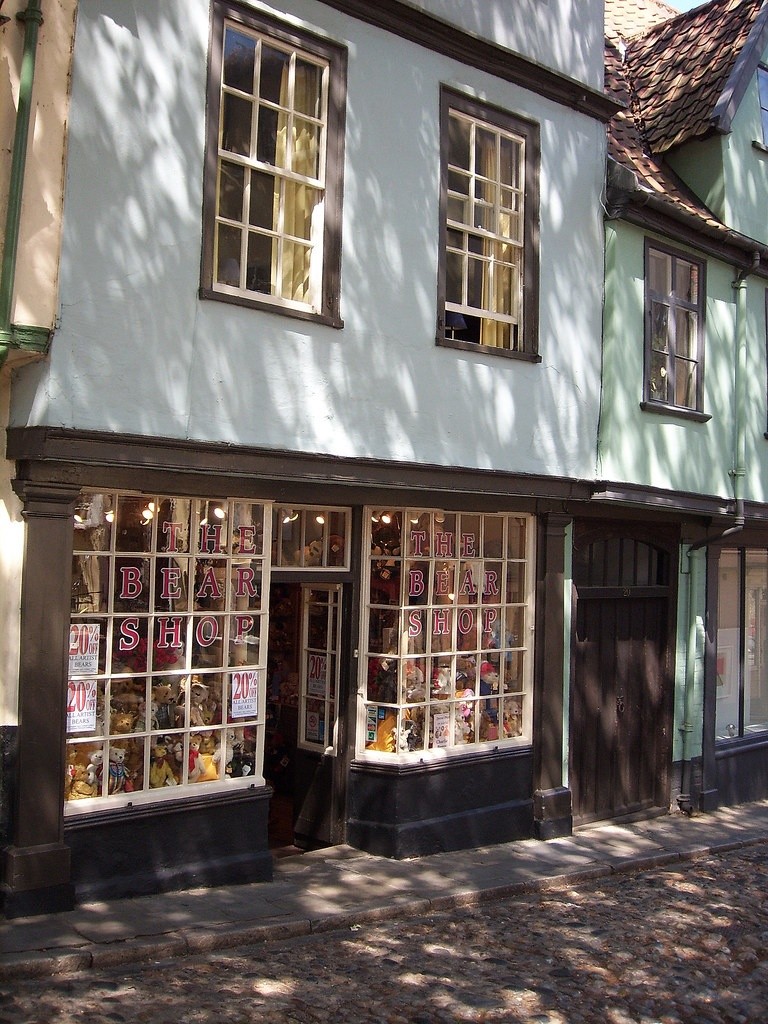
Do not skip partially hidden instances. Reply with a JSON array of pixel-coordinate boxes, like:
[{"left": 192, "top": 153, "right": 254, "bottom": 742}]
[
  {"left": 394, "top": 656, "right": 521, "bottom": 754},
  {"left": 45, "top": 641, "right": 257, "bottom": 801}
]
